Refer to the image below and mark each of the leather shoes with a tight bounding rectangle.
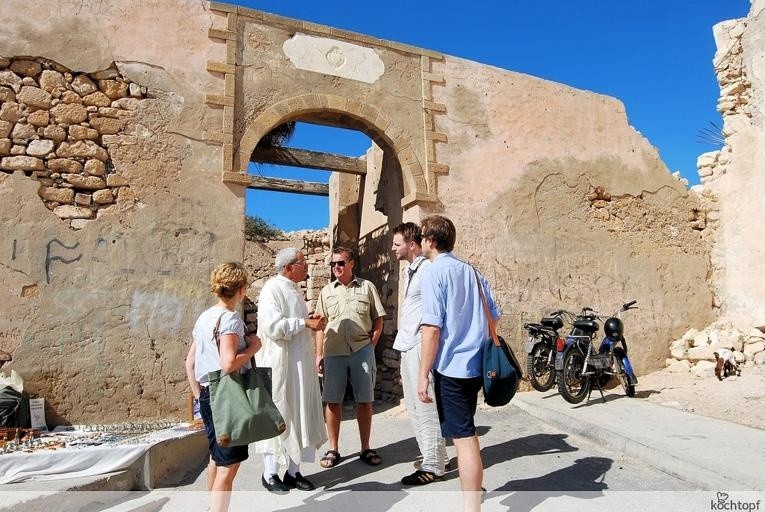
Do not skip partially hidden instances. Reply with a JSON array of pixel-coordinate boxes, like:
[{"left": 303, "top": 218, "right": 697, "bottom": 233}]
[
  {"left": 261, "top": 472, "right": 291, "bottom": 497},
  {"left": 282, "top": 469, "right": 316, "bottom": 492}
]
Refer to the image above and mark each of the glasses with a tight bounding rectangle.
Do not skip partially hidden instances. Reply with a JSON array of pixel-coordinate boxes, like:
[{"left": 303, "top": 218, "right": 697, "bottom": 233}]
[
  {"left": 329, "top": 258, "right": 349, "bottom": 268},
  {"left": 289, "top": 260, "right": 307, "bottom": 267},
  {"left": 418, "top": 231, "right": 427, "bottom": 239}
]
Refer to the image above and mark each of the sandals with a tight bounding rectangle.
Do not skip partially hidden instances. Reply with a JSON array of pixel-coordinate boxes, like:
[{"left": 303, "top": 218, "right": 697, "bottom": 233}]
[
  {"left": 319, "top": 449, "right": 342, "bottom": 469},
  {"left": 359, "top": 448, "right": 384, "bottom": 468}
]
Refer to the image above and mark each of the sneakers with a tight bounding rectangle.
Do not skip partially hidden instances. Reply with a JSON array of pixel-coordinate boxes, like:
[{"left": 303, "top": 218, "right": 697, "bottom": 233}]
[
  {"left": 479, "top": 487, "right": 489, "bottom": 505},
  {"left": 413, "top": 459, "right": 452, "bottom": 472},
  {"left": 400, "top": 469, "right": 447, "bottom": 486}
]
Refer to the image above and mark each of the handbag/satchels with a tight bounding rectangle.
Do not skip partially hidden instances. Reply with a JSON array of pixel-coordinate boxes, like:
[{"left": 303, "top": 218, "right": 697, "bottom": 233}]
[
  {"left": 480, "top": 332, "right": 532, "bottom": 409},
  {"left": 206, "top": 311, "right": 288, "bottom": 449}
]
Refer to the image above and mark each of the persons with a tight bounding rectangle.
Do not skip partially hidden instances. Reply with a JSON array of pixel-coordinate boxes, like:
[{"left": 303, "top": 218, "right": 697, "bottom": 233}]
[
  {"left": 391, "top": 222, "right": 452, "bottom": 485},
  {"left": 185, "top": 261, "right": 286, "bottom": 512},
  {"left": 315, "top": 246, "right": 387, "bottom": 468},
  {"left": 255, "top": 248, "right": 328, "bottom": 495},
  {"left": 417, "top": 215, "right": 522, "bottom": 512}
]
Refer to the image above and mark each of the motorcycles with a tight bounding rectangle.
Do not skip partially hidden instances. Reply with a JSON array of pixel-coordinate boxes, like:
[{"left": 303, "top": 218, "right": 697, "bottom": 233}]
[
  {"left": 554, "top": 298, "right": 642, "bottom": 407},
  {"left": 524, "top": 305, "right": 606, "bottom": 393}
]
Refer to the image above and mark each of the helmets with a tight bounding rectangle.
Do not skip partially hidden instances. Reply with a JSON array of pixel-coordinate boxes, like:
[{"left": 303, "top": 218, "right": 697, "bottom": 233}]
[{"left": 603, "top": 316, "right": 624, "bottom": 343}]
